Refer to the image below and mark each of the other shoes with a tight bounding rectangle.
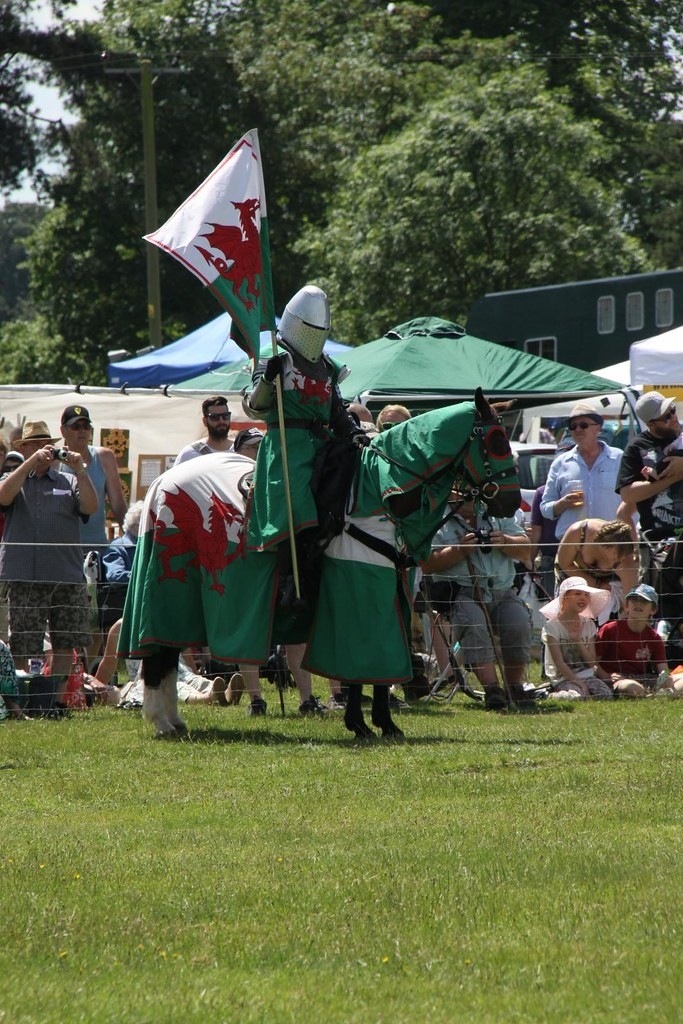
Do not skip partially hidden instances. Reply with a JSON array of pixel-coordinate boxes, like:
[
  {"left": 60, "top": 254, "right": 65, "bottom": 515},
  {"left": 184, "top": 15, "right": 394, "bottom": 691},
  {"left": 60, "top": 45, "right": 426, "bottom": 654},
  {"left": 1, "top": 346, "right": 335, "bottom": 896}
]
[
  {"left": 505, "top": 684, "right": 534, "bottom": 710},
  {"left": 46, "top": 701, "right": 68, "bottom": 720},
  {"left": 651, "top": 687, "right": 674, "bottom": 697},
  {"left": 533, "top": 683, "right": 553, "bottom": 699},
  {"left": 483, "top": 685, "right": 509, "bottom": 710},
  {"left": 210, "top": 673, "right": 411, "bottom": 717}
]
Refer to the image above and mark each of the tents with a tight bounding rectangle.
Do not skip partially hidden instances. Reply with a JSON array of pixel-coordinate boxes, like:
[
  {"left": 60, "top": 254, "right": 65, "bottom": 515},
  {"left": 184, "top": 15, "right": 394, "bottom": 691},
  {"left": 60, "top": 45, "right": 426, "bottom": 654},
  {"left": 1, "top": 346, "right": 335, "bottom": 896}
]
[
  {"left": 163, "top": 308, "right": 621, "bottom": 404},
  {"left": 108, "top": 308, "right": 357, "bottom": 393}
]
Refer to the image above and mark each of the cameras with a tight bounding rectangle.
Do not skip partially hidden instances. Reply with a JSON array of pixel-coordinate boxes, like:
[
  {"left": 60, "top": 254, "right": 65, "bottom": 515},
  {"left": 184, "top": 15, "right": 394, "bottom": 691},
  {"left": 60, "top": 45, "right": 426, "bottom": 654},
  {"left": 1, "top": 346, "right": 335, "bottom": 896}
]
[
  {"left": 473, "top": 528, "right": 493, "bottom": 554},
  {"left": 52, "top": 448, "right": 69, "bottom": 460}
]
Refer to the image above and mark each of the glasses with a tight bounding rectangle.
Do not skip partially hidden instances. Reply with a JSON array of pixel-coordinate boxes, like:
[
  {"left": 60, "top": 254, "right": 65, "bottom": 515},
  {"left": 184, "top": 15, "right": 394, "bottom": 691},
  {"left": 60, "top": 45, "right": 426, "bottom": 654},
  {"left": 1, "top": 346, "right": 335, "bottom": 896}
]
[
  {"left": 64, "top": 423, "right": 91, "bottom": 430},
  {"left": 569, "top": 421, "right": 599, "bottom": 430},
  {"left": 207, "top": 412, "right": 231, "bottom": 421},
  {"left": 380, "top": 421, "right": 403, "bottom": 432},
  {"left": 651, "top": 404, "right": 677, "bottom": 423},
  {"left": 3, "top": 464, "right": 20, "bottom": 472},
  {"left": 239, "top": 444, "right": 259, "bottom": 452}
]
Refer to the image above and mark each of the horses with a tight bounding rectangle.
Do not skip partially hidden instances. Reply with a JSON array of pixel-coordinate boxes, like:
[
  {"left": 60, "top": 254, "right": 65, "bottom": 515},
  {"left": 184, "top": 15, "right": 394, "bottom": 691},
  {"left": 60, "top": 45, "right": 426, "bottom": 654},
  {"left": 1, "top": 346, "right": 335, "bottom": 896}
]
[{"left": 145, "top": 385, "right": 520, "bottom": 741}]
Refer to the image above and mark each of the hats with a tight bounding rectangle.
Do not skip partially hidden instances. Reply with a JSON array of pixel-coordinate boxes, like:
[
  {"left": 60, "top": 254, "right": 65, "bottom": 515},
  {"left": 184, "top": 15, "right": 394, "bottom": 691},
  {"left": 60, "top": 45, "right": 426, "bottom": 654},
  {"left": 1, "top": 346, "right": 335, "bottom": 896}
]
[
  {"left": 538, "top": 576, "right": 611, "bottom": 620},
  {"left": 635, "top": 391, "right": 677, "bottom": 422},
  {"left": 570, "top": 404, "right": 598, "bottom": 418},
  {"left": 624, "top": 584, "right": 658, "bottom": 607},
  {"left": 234, "top": 427, "right": 264, "bottom": 451},
  {"left": 13, "top": 421, "right": 62, "bottom": 449},
  {"left": 5, "top": 451, "right": 25, "bottom": 463},
  {"left": 62, "top": 405, "right": 91, "bottom": 427}
]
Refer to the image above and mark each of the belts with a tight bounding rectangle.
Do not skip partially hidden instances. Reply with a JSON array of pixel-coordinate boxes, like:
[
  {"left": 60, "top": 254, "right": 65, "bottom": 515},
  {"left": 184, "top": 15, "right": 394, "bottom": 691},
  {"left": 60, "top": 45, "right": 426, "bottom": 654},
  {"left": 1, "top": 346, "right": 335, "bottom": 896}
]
[{"left": 268, "top": 418, "right": 330, "bottom": 438}]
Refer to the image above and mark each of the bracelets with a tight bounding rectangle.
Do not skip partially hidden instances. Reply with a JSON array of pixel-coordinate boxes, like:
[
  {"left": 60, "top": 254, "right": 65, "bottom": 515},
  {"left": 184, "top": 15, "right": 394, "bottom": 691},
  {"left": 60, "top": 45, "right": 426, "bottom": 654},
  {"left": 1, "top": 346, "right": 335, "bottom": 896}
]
[
  {"left": 75, "top": 469, "right": 84, "bottom": 474},
  {"left": 502, "top": 535, "right": 506, "bottom": 551}
]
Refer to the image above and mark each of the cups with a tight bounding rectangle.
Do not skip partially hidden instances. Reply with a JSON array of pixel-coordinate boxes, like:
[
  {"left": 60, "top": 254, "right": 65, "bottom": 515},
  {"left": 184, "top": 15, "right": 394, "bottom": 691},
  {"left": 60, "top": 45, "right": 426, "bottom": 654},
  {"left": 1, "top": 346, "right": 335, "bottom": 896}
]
[{"left": 569, "top": 480, "right": 584, "bottom": 505}]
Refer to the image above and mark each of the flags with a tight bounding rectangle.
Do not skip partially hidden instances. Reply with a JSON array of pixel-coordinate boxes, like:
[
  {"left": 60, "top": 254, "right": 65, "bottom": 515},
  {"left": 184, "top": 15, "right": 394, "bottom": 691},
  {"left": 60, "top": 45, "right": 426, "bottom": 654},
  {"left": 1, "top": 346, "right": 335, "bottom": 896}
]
[{"left": 144, "top": 131, "right": 278, "bottom": 376}]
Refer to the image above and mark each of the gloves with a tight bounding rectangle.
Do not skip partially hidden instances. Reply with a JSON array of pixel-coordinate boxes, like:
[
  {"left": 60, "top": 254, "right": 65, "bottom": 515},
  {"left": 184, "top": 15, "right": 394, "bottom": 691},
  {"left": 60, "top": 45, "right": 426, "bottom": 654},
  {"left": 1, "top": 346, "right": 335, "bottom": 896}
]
[
  {"left": 265, "top": 355, "right": 284, "bottom": 381},
  {"left": 352, "top": 432, "right": 371, "bottom": 450}
]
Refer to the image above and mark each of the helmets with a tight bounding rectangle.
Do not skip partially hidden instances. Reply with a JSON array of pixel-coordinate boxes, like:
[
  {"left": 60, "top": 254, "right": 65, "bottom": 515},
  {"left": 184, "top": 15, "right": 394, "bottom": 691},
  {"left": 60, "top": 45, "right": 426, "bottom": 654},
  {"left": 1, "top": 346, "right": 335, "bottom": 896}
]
[{"left": 277, "top": 284, "right": 331, "bottom": 362}]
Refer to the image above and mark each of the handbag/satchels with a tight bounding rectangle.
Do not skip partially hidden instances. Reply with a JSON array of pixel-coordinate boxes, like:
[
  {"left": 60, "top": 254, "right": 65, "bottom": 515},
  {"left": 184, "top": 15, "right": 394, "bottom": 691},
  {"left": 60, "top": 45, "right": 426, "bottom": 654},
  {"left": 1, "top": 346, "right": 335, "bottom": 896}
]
[{"left": 414, "top": 580, "right": 461, "bottom": 612}]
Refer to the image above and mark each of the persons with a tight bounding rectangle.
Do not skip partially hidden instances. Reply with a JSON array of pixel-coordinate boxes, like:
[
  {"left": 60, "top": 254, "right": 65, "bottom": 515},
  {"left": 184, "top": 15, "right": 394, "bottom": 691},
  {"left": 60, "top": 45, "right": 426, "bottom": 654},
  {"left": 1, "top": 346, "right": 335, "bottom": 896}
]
[
  {"left": 329, "top": 389, "right": 682, "bottom": 706},
  {"left": 0, "top": 397, "right": 331, "bottom": 718},
  {"left": 241, "top": 285, "right": 372, "bottom": 611}
]
